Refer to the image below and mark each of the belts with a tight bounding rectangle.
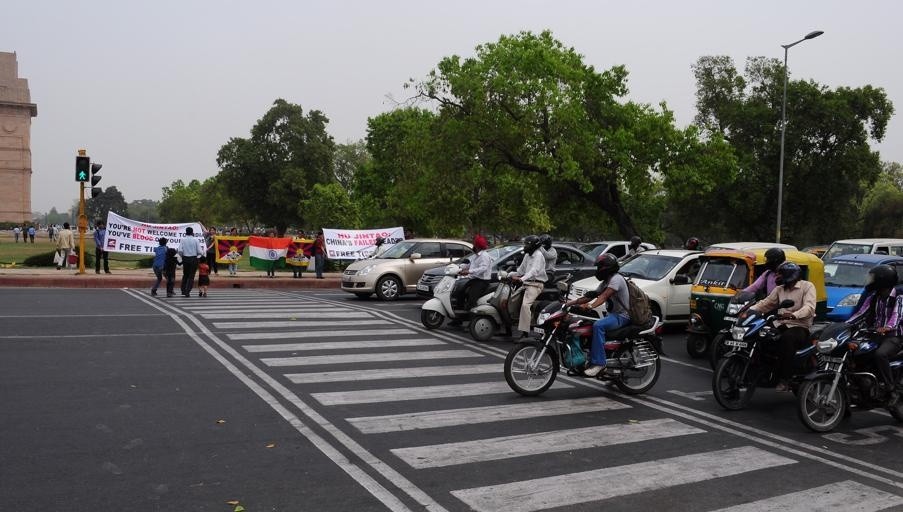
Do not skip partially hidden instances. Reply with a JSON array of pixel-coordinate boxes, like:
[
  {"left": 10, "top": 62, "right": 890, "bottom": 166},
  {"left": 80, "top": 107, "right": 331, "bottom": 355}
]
[{"left": 525, "top": 279, "right": 546, "bottom": 283}]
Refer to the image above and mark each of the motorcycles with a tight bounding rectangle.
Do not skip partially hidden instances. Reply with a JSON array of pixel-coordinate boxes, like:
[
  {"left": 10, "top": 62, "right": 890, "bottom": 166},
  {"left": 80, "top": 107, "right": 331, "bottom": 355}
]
[
  {"left": 686, "top": 250, "right": 829, "bottom": 356},
  {"left": 706, "top": 280, "right": 903, "bottom": 437},
  {"left": 421, "top": 253, "right": 503, "bottom": 331},
  {"left": 504, "top": 276, "right": 671, "bottom": 402},
  {"left": 469, "top": 260, "right": 569, "bottom": 348}
]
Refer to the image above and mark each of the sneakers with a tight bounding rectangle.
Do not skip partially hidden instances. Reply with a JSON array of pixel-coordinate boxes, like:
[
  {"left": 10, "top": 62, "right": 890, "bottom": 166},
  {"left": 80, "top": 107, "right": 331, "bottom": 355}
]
[
  {"left": 95, "top": 270, "right": 112, "bottom": 275},
  {"left": 151, "top": 290, "right": 207, "bottom": 298},
  {"left": 584, "top": 364, "right": 608, "bottom": 377}
]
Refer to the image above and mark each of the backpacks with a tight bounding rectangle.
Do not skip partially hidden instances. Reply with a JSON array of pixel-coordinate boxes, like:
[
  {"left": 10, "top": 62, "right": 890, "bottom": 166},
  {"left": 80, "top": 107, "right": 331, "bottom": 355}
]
[{"left": 614, "top": 277, "right": 651, "bottom": 324}]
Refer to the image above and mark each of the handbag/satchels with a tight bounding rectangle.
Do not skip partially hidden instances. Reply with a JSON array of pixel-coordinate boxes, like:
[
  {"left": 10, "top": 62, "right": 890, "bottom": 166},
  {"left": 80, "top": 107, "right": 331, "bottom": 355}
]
[
  {"left": 68, "top": 253, "right": 78, "bottom": 266},
  {"left": 53, "top": 250, "right": 61, "bottom": 264}
]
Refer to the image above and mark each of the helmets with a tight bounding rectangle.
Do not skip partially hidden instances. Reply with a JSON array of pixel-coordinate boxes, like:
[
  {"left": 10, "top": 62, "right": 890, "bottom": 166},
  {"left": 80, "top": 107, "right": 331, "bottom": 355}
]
[
  {"left": 375, "top": 237, "right": 383, "bottom": 245},
  {"left": 686, "top": 237, "right": 699, "bottom": 249},
  {"left": 864, "top": 263, "right": 899, "bottom": 293},
  {"left": 774, "top": 262, "right": 802, "bottom": 287},
  {"left": 539, "top": 233, "right": 554, "bottom": 247},
  {"left": 763, "top": 247, "right": 786, "bottom": 268},
  {"left": 593, "top": 253, "right": 620, "bottom": 281},
  {"left": 631, "top": 235, "right": 642, "bottom": 245},
  {"left": 523, "top": 235, "right": 540, "bottom": 252}
]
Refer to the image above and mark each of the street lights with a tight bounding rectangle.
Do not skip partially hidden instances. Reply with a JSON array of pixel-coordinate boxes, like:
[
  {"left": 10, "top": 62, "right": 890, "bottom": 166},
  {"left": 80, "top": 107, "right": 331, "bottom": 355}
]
[{"left": 770, "top": 33, "right": 831, "bottom": 248}]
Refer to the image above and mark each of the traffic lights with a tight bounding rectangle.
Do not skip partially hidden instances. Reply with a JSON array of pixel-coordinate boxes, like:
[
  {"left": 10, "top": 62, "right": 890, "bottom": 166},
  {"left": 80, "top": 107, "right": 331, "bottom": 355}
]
[
  {"left": 91, "top": 164, "right": 103, "bottom": 199},
  {"left": 75, "top": 156, "right": 91, "bottom": 183}
]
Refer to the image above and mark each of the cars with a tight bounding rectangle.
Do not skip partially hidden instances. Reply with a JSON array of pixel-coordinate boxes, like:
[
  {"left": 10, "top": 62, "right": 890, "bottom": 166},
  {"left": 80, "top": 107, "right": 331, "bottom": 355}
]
[
  {"left": 415, "top": 242, "right": 599, "bottom": 300},
  {"left": 339, "top": 238, "right": 488, "bottom": 302}
]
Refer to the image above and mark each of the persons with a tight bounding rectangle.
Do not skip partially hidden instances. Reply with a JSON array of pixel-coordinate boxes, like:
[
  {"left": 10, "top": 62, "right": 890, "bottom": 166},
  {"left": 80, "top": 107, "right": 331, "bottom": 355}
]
[
  {"left": 13, "top": 221, "right": 78, "bottom": 270},
  {"left": 93, "top": 219, "right": 112, "bottom": 274},
  {"left": 367, "top": 229, "right": 414, "bottom": 260},
  {"left": 151, "top": 222, "right": 329, "bottom": 297}
]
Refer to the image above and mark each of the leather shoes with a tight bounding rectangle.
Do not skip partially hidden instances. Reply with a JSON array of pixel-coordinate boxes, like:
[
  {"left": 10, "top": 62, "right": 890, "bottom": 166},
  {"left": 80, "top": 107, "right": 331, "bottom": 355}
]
[{"left": 775, "top": 381, "right": 790, "bottom": 393}]
